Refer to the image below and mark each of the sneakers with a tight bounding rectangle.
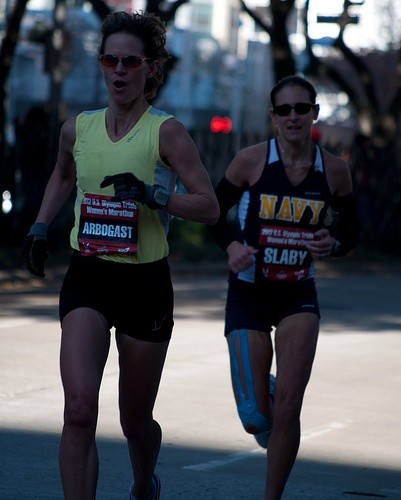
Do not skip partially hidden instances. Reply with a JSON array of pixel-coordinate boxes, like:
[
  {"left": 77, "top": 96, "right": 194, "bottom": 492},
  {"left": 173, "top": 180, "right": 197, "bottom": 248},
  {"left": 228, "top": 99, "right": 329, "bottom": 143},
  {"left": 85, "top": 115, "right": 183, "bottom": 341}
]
[
  {"left": 254, "top": 375, "right": 276, "bottom": 448},
  {"left": 129, "top": 474, "right": 161, "bottom": 500}
]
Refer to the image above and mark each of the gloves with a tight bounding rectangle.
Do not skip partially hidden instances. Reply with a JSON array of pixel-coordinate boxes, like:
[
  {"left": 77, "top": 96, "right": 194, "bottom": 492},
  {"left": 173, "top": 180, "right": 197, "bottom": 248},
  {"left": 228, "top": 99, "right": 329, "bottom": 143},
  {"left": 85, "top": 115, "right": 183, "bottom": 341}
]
[
  {"left": 100, "top": 173, "right": 155, "bottom": 210},
  {"left": 26, "top": 223, "right": 49, "bottom": 277}
]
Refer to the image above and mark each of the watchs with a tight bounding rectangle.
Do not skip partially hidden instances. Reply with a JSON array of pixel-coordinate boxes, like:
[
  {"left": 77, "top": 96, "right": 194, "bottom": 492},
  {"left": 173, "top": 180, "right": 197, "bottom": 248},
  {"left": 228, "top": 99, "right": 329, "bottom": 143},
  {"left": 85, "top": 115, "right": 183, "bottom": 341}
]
[
  {"left": 330, "top": 235, "right": 342, "bottom": 257},
  {"left": 148, "top": 184, "right": 170, "bottom": 211}
]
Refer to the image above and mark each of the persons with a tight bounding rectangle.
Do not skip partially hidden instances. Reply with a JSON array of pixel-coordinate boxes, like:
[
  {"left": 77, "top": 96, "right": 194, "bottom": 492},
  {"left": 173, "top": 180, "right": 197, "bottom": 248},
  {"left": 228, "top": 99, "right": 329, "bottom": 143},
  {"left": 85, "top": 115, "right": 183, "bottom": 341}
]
[
  {"left": 204, "top": 76, "right": 358, "bottom": 500},
  {"left": 22, "top": 11, "right": 220, "bottom": 500}
]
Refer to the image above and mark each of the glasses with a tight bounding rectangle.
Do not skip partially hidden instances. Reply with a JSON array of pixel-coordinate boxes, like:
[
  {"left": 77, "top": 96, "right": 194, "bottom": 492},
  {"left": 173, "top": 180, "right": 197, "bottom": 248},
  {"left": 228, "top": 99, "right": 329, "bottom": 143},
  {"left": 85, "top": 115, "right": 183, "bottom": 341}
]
[
  {"left": 97, "top": 54, "right": 150, "bottom": 67},
  {"left": 273, "top": 102, "right": 314, "bottom": 116}
]
